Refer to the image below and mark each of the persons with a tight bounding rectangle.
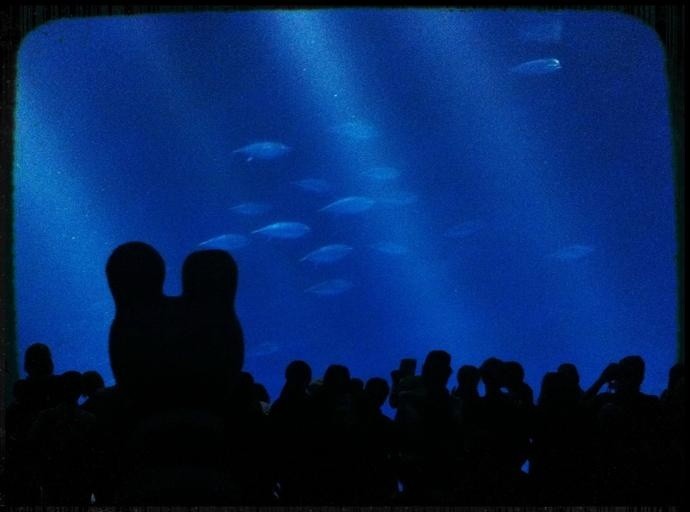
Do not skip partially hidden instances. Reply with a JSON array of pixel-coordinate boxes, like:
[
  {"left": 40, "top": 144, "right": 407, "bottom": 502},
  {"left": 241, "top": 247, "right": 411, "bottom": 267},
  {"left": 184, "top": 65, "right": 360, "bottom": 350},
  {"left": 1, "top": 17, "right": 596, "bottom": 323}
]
[
  {"left": 104, "top": 242, "right": 191, "bottom": 512},
  {"left": 173, "top": 246, "right": 261, "bottom": 511}
]
